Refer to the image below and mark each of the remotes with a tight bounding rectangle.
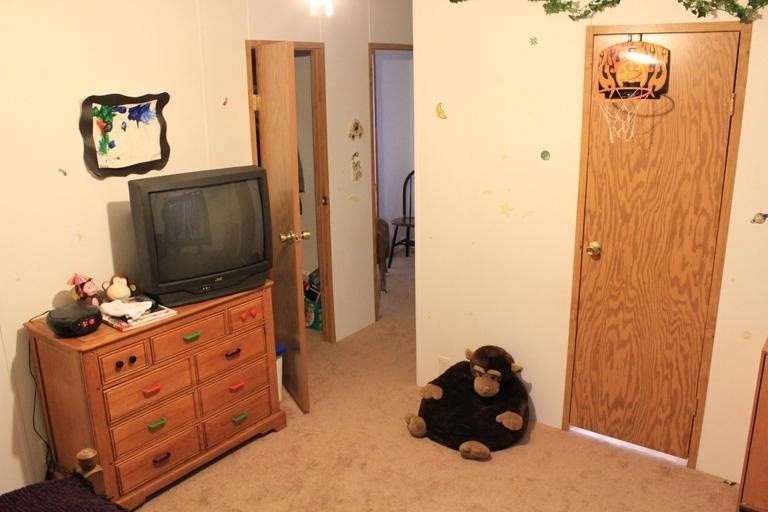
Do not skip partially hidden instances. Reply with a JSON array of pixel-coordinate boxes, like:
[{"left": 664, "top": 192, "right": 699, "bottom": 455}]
[{"left": 135, "top": 296, "right": 157, "bottom": 310}]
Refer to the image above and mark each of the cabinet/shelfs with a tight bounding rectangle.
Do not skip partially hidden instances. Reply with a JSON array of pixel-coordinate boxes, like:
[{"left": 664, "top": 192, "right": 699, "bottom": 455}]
[
  {"left": 23, "top": 269, "right": 288, "bottom": 512},
  {"left": 735, "top": 334, "right": 768, "bottom": 512}
]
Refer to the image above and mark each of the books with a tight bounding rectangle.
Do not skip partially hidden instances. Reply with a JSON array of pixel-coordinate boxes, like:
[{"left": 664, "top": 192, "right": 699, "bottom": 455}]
[{"left": 93, "top": 293, "right": 178, "bottom": 332}]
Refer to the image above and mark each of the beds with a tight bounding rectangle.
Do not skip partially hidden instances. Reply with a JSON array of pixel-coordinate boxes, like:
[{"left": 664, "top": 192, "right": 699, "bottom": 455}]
[{"left": 0, "top": 448, "right": 131, "bottom": 512}]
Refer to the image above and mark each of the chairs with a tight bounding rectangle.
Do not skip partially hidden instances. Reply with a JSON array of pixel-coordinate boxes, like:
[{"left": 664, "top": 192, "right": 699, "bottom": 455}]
[{"left": 387, "top": 169, "right": 415, "bottom": 269}]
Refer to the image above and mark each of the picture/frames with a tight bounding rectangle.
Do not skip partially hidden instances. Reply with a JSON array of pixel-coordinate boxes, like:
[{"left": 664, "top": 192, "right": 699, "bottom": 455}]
[{"left": 78, "top": 92, "right": 172, "bottom": 179}]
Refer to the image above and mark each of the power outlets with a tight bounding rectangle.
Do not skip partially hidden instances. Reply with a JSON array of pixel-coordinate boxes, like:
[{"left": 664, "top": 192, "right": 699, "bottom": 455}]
[{"left": 40, "top": 440, "right": 52, "bottom": 464}]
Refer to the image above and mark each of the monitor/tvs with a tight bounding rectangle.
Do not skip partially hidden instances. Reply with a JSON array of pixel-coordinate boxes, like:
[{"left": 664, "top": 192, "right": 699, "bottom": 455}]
[{"left": 128, "top": 161, "right": 274, "bottom": 306}]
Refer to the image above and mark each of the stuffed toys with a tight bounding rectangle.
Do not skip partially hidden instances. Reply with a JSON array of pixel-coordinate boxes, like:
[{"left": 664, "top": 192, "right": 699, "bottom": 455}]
[
  {"left": 74, "top": 273, "right": 103, "bottom": 308},
  {"left": 102, "top": 273, "right": 138, "bottom": 300},
  {"left": 405, "top": 345, "right": 530, "bottom": 462}
]
